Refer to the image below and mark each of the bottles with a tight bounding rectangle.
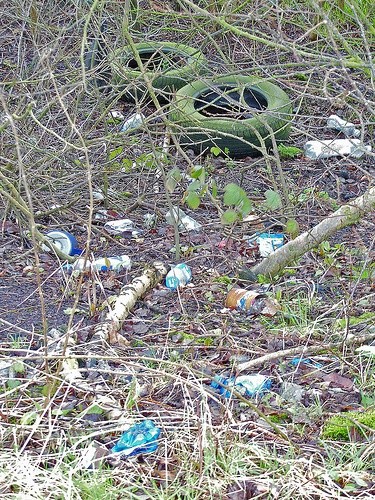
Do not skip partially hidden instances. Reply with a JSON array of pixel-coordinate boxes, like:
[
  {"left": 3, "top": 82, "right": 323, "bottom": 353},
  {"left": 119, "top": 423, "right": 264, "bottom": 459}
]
[
  {"left": 61, "top": 255, "right": 131, "bottom": 273},
  {"left": 327, "top": 114, "right": 361, "bottom": 137},
  {"left": 165, "top": 263, "right": 191, "bottom": 288}
]
